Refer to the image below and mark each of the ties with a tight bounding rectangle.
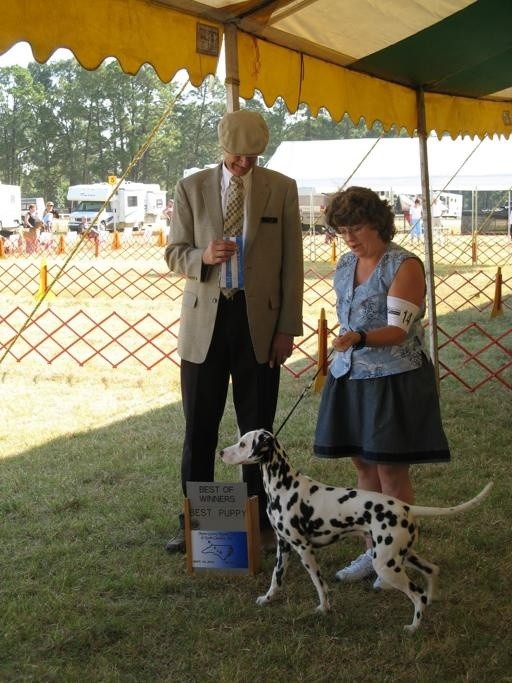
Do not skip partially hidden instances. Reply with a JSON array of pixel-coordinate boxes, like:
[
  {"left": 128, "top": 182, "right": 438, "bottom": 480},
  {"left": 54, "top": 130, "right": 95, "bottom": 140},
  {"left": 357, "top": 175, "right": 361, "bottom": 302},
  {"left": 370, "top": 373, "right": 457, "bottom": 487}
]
[{"left": 218, "top": 175, "right": 244, "bottom": 300}]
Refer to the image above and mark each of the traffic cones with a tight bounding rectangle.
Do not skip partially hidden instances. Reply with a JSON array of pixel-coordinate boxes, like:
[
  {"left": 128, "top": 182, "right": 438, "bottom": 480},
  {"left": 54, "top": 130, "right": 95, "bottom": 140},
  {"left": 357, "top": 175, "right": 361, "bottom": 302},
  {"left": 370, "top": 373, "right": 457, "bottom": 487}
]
[
  {"left": 55, "top": 234, "right": 69, "bottom": 253},
  {"left": 153, "top": 227, "right": 167, "bottom": 247},
  {"left": 108, "top": 230, "right": 123, "bottom": 249}
]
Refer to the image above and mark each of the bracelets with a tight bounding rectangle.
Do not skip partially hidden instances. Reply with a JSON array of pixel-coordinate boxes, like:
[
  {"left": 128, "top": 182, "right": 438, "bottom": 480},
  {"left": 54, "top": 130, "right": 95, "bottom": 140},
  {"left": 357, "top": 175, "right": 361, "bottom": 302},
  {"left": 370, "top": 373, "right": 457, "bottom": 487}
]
[{"left": 352, "top": 329, "right": 367, "bottom": 350}]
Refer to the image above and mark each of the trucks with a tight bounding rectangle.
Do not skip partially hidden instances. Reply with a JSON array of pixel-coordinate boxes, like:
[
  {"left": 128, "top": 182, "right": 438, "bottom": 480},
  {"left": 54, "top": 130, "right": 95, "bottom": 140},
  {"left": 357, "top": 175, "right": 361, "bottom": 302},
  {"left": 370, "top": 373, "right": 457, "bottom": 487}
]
[
  {"left": 21, "top": 197, "right": 45, "bottom": 224},
  {"left": 63, "top": 178, "right": 169, "bottom": 233}
]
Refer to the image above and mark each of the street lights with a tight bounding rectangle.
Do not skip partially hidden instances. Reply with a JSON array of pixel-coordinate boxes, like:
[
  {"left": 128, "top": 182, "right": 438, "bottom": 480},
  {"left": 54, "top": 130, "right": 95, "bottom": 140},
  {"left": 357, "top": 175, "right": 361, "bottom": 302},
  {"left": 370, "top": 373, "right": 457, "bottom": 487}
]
[{"left": 257, "top": 155, "right": 265, "bottom": 166}]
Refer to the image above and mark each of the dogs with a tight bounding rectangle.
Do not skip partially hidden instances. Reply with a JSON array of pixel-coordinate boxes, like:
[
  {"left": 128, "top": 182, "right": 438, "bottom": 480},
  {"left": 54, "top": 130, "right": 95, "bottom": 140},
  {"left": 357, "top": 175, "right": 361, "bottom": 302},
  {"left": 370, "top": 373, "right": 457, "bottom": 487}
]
[{"left": 218, "top": 429, "right": 495, "bottom": 634}]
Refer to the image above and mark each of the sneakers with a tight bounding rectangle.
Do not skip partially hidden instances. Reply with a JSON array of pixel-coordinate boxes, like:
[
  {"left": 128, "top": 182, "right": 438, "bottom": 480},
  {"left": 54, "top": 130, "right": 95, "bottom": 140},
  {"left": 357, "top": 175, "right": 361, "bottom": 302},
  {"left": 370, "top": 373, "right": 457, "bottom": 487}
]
[
  {"left": 334, "top": 548, "right": 376, "bottom": 582},
  {"left": 372, "top": 576, "right": 393, "bottom": 592}
]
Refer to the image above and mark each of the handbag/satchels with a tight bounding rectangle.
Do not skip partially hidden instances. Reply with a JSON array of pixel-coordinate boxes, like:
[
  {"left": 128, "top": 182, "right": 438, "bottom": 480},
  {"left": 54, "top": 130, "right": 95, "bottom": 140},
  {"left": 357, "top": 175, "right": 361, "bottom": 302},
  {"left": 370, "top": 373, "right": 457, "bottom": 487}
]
[{"left": 27, "top": 216, "right": 35, "bottom": 228}]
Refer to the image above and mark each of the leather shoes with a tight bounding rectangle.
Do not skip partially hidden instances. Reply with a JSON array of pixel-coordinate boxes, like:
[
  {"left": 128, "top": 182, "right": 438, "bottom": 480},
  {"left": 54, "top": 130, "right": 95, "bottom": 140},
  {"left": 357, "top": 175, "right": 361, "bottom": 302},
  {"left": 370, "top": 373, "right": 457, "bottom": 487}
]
[{"left": 166, "top": 517, "right": 199, "bottom": 550}]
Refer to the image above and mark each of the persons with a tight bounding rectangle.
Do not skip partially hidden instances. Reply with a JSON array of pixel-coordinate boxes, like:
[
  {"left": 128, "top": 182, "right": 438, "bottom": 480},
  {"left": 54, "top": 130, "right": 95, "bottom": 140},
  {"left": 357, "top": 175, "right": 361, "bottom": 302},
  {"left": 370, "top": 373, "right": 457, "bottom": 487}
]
[
  {"left": 24, "top": 202, "right": 38, "bottom": 228},
  {"left": 165, "top": 108, "right": 305, "bottom": 552},
  {"left": 42, "top": 200, "right": 54, "bottom": 218},
  {"left": 431, "top": 193, "right": 449, "bottom": 246},
  {"left": 312, "top": 184, "right": 450, "bottom": 583},
  {"left": 40, "top": 209, "right": 59, "bottom": 234},
  {"left": 78, "top": 216, "right": 100, "bottom": 237},
  {"left": 410, "top": 198, "right": 423, "bottom": 246},
  {"left": 162, "top": 198, "right": 174, "bottom": 226}
]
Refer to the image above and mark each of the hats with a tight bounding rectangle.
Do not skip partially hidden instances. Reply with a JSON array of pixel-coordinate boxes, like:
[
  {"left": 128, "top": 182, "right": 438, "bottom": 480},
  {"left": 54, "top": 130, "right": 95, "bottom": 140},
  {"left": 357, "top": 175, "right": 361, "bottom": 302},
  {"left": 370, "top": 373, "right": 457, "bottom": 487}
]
[
  {"left": 46, "top": 201, "right": 54, "bottom": 206},
  {"left": 217, "top": 111, "right": 269, "bottom": 156}
]
